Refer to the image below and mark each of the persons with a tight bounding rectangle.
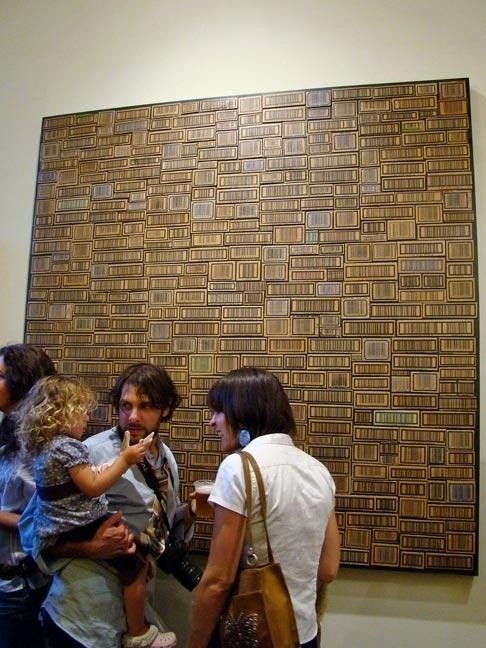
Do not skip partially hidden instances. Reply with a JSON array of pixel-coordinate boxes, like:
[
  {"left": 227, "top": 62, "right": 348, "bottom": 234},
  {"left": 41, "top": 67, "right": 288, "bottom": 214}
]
[
  {"left": 38, "top": 362, "right": 199, "bottom": 646},
  {"left": 12, "top": 375, "right": 179, "bottom": 647},
  {"left": 184, "top": 367, "right": 341, "bottom": 647},
  {"left": 0, "top": 344, "right": 59, "bottom": 647}
]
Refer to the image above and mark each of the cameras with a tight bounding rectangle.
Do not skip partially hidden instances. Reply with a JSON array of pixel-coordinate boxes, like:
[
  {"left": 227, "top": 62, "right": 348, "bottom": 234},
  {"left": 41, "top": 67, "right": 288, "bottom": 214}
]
[{"left": 155, "top": 538, "right": 205, "bottom": 592}]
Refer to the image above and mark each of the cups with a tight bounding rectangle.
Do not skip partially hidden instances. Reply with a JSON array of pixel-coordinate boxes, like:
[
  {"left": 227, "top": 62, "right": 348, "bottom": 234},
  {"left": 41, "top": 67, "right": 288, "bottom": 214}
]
[{"left": 193, "top": 479, "right": 217, "bottom": 520}]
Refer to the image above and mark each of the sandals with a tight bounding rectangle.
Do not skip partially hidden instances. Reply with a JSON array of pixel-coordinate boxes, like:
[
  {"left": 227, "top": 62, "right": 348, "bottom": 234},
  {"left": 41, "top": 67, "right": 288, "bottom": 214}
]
[{"left": 122, "top": 623, "right": 177, "bottom": 648}]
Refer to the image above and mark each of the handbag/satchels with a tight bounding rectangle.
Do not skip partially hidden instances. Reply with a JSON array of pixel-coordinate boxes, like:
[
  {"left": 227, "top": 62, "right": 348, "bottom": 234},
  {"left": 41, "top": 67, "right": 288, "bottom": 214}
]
[{"left": 218, "top": 449, "right": 301, "bottom": 648}]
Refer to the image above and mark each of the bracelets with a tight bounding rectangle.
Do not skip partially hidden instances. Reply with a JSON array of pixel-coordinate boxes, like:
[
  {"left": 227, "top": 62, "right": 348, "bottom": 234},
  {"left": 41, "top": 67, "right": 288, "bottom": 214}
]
[{"left": 187, "top": 502, "right": 197, "bottom": 519}]
